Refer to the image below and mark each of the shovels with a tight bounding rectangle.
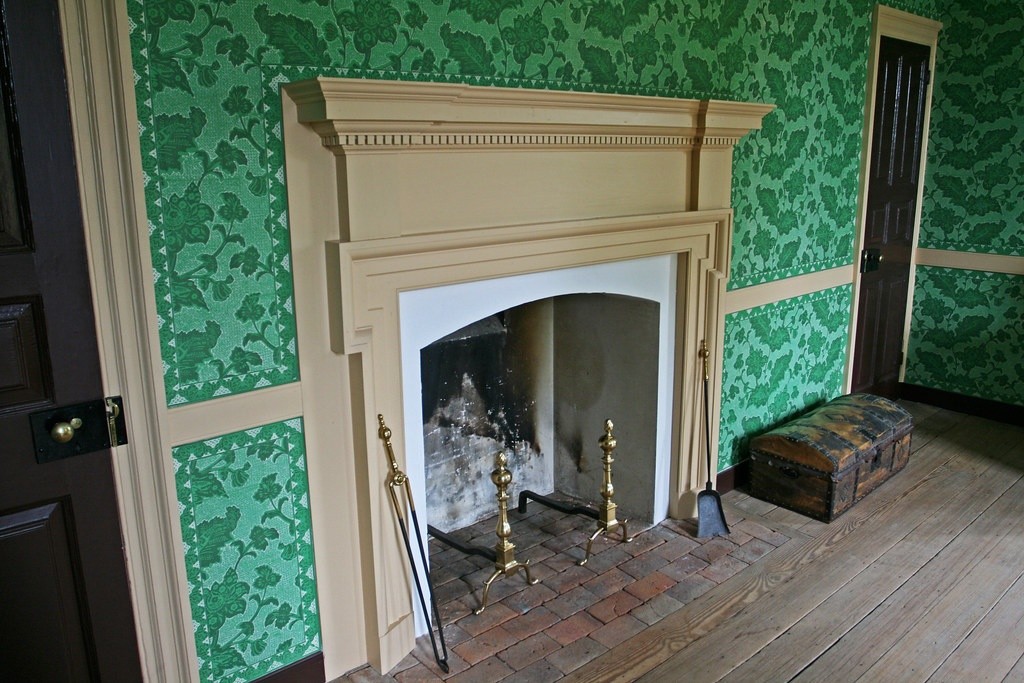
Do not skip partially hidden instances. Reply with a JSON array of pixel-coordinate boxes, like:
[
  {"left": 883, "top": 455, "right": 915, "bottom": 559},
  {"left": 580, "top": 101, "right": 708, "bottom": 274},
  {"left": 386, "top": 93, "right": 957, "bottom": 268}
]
[{"left": 695, "top": 340, "right": 732, "bottom": 539}]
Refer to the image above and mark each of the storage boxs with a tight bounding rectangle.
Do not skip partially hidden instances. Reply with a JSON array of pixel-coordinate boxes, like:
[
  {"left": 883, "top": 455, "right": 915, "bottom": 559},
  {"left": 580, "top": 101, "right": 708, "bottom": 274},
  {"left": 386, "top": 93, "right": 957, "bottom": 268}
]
[{"left": 746, "top": 392, "right": 914, "bottom": 524}]
